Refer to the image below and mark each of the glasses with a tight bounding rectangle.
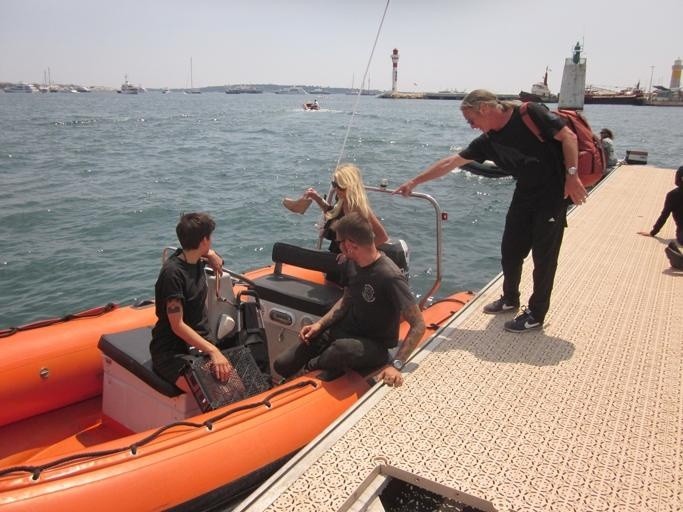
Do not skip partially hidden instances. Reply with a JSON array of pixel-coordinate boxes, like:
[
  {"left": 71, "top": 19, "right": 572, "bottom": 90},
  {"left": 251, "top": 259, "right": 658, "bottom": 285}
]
[{"left": 332, "top": 182, "right": 345, "bottom": 191}]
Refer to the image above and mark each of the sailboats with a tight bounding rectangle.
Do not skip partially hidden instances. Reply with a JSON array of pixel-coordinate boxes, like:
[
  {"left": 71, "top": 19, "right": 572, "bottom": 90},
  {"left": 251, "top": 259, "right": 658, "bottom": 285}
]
[
  {"left": 359, "top": 72, "right": 378, "bottom": 96},
  {"left": 38, "top": 69, "right": 49, "bottom": 94},
  {"left": 183, "top": 56, "right": 201, "bottom": 95},
  {"left": 48, "top": 67, "right": 59, "bottom": 93},
  {"left": 343, "top": 71, "right": 361, "bottom": 96}
]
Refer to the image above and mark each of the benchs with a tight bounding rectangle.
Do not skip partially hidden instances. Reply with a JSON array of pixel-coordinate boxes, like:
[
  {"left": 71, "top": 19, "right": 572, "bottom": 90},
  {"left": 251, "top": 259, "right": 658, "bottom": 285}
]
[{"left": 248, "top": 242, "right": 341, "bottom": 332}]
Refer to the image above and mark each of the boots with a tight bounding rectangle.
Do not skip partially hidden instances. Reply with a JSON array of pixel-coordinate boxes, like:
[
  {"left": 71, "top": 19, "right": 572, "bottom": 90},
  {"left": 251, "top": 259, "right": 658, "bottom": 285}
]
[{"left": 283, "top": 198, "right": 312, "bottom": 214}]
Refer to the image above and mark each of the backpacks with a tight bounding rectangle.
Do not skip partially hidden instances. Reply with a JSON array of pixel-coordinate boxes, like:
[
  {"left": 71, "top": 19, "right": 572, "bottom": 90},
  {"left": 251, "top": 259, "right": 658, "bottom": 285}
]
[{"left": 519, "top": 102, "right": 607, "bottom": 188}]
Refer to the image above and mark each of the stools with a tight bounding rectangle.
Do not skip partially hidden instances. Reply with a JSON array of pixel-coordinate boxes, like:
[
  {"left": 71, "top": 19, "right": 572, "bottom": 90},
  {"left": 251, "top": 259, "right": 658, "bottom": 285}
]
[{"left": 98, "top": 324, "right": 200, "bottom": 436}]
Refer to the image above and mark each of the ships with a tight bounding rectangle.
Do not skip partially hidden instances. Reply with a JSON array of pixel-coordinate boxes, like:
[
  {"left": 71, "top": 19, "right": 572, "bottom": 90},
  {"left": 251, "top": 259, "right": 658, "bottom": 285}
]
[
  {"left": 517, "top": 65, "right": 559, "bottom": 104},
  {"left": 307, "top": 88, "right": 333, "bottom": 95},
  {"left": 75, "top": 85, "right": 92, "bottom": 93},
  {"left": 224, "top": 81, "right": 264, "bottom": 95},
  {"left": 584, "top": 86, "right": 649, "bottom": 107},
  {"left": 270, "top": 84, "right": 310, "bottom": 95}
]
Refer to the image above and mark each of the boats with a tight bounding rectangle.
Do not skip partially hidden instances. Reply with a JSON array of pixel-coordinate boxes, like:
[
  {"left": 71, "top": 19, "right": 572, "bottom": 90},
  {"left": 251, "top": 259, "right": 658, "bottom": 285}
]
[
  {"left": 115, "top": 72, "right": 145, "bottom": 94},
  {"left": 301, "top": 102, "right": 320, "bottom": 112},
  {"left": 604, "top": 148, "right": 650, "bottom": 178},
  {"left": 162, "top": 88, "right": 169, "bottom": 95},
  {"left": 458, "top": 158, "right": 514, "bottom": 179},
  {"left": 0, "top": 184, "right": 478, "bottom": 511},
  {"left": 2, "top": 82, "right": 40, "bottom": 94}
]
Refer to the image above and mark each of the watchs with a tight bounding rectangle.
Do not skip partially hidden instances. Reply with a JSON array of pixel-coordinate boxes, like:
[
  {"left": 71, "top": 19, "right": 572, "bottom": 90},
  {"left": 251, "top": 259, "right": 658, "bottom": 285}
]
[
  {"left": 566, "top": 166, "right": 577, "bottom": 176},
  {"left": 390, "top": 358, "right": 404, "bottom": 370}
]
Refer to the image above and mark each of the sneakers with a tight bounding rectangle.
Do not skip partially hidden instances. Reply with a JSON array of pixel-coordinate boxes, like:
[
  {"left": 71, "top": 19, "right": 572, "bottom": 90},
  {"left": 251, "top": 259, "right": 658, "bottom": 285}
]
[
  {"left": 484, "top": 295, "right": 519, "bottom": 314},
  {"left": 503, "top": 305, "right": 543, "bottom": 332}
]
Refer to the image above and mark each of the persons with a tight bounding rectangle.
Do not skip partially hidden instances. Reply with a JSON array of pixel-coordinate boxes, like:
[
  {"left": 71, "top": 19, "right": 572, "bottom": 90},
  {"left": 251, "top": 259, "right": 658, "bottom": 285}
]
[
  {"left": 149, "top": 211, "right": 232, "bottom": 399},
  {"left": 272, "top": 211, "right": 426, "bottom": 388},
  {"left": 301, "top": 161, "right": 388, "bottom": 289},
  {"left": 390, "top": 88, "right": 589, "bottom": 334},
  {"left": 636, "top": 165, "right": 683, "bottom": 270},
  {"left": 314, "top": 99, "right": 318, "bottom": 105},
  {"left": 599, "top": 128, "right": 618, "bottom": 167}
]
